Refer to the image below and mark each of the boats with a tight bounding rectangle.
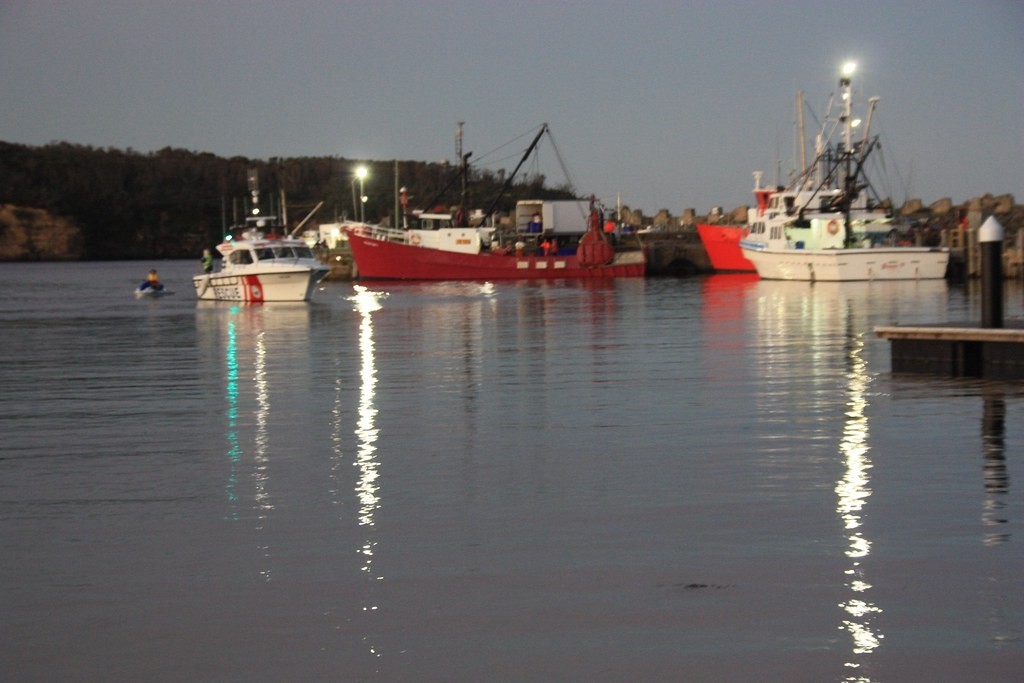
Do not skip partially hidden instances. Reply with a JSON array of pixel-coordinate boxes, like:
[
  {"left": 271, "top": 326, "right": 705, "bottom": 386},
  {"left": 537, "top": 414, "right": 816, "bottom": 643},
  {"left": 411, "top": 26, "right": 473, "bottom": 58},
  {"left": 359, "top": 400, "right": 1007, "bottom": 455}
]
[{"left": 135, "top": 58, "right": 961, "bottom": 305}]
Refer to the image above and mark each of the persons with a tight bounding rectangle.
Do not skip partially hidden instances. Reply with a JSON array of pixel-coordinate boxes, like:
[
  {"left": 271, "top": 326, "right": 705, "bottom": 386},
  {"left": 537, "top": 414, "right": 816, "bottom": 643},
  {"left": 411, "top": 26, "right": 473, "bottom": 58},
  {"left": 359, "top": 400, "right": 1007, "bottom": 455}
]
[
  {"left": 146, "top": 268, "right": 160, "bottom": 290},
  {"left": 490, "top": 234, "right": 559, "bottom": 257},
  {"left": 200, "top": 249, "right": 213, "bottom": 273}
]
